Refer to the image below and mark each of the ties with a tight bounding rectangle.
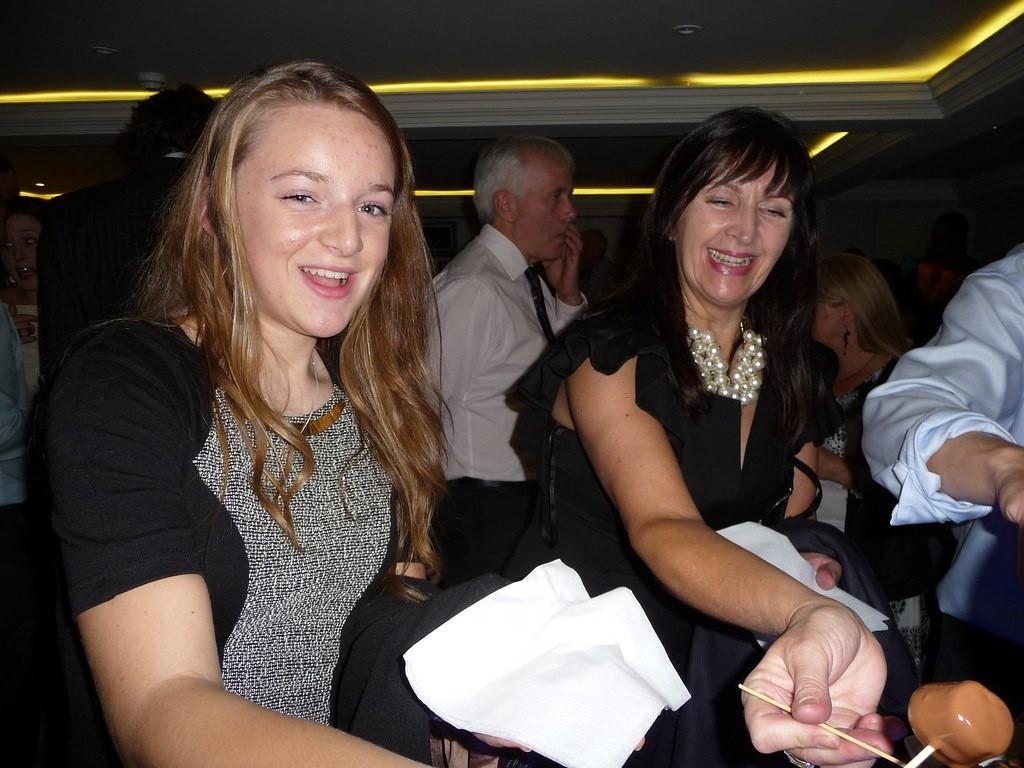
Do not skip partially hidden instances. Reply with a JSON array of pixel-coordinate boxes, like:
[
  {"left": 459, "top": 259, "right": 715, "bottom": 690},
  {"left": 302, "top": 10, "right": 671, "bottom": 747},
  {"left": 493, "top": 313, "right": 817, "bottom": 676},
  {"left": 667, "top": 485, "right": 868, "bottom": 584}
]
[{"left": 523, "top": 266, "right": 556, "bottom": 343}]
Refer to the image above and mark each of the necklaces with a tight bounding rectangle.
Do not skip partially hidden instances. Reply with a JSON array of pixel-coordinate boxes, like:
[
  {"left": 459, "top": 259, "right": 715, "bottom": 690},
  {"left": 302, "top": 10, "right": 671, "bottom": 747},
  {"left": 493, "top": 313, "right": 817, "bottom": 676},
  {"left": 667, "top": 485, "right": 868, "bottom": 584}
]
[
  {"left": 685, "top": 319, "right": 767, "bottom": 409},
  {"left": 839, "top": 353, "right": 876, "bottom": 382},
  {"left": 297, "top": 354, "right": 320, "bottom": 435}
]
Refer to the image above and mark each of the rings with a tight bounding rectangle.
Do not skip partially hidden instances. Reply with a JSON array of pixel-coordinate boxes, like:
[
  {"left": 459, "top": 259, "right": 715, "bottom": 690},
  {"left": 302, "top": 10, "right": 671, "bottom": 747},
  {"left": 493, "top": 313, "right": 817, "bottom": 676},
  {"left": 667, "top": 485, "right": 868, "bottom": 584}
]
[
  {"left": 783, "top": 750, "right": 814, "bottom": 768},
  {"left": 17, "top": 329, "right": 23, "bottom": 338}
]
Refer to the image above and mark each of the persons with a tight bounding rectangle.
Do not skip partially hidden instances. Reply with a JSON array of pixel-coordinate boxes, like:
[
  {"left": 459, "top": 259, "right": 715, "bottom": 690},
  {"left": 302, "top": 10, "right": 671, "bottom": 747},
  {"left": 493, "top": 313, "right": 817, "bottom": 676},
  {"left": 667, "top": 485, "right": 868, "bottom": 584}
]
[
  {"left": 811, "top": 245, "right": 960, "bottom": 686},
  {"left": 505, "top": 103, "right": 911, "bottom": 768},
  {"left": 419, "top": 133, "right": 588, "bottom": 595},
  {"left": 578, "top": 227, "right": 618, "bottom": 298},
  {"left": 35, "top": 86, "right": 216, "bottom": 376},
  {"left": 0, "top": 194, "right": 45, "bottom": 768},
  {"left": 858, "top": 239, "right": 1024, "bottom": 760},
  {"left": 0, "top": 57, "right": 645, "bottom": 768}
]
